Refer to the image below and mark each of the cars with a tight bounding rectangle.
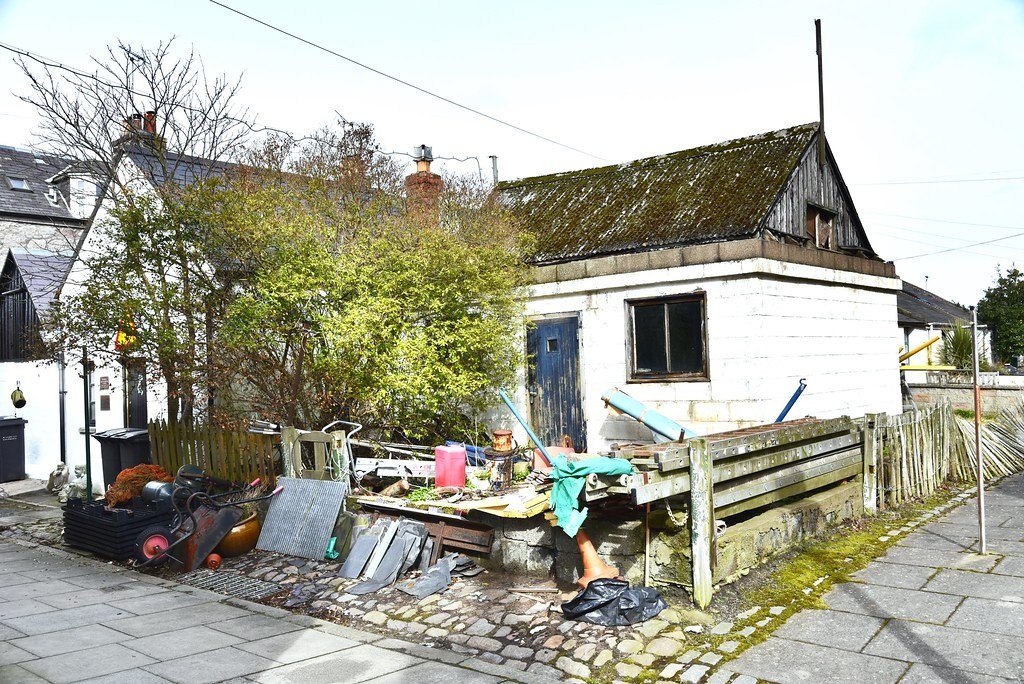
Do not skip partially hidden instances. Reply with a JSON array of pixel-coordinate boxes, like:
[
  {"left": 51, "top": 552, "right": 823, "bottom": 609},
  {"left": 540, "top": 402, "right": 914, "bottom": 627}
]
[{"left": 1004, "top": 365, "right": 1017, "bottom": 374}]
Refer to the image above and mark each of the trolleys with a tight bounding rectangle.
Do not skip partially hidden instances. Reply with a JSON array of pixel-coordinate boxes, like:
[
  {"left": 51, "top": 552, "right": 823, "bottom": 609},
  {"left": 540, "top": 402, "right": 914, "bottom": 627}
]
[{"left": 132, "top": 473, "right": 285, "bottom": 574}]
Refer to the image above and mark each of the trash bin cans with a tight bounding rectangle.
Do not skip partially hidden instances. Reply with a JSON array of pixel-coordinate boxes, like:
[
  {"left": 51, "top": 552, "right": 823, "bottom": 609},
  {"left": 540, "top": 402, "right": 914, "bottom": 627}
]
[
  {"left": 91, "top": 428, "right": 149, "bottom": 494},
  {"left": 0, "top": 416, "right": 28, "bottom": 483}
]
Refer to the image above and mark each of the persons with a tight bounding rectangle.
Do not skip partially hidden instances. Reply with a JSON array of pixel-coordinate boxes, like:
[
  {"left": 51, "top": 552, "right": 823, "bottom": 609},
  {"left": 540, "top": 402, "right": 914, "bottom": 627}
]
[
  {"left": 52, "top": 461, "right": 68, "bottom": 492},
  {"left": 63, "top": 464, "right": 88, "bottom": 501}
]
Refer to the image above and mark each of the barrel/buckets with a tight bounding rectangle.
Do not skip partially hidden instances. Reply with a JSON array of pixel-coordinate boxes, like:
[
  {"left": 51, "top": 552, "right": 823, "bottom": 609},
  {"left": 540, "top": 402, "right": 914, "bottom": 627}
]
[
  {"left": 435, "top": 441, "right": 466, "bottom": 489},
  {"left": 484, "top": 448, "right": 515, "bottom": 488},
  {"left": 493, "top": 429, "right": 513, "bottom": 452}
]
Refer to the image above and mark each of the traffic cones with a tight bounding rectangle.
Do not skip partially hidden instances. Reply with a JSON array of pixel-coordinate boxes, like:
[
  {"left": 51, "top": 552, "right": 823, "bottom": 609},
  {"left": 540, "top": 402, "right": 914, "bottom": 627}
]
[{"left": 576, "top": 530, "right": 619, "bottom": 588}]
